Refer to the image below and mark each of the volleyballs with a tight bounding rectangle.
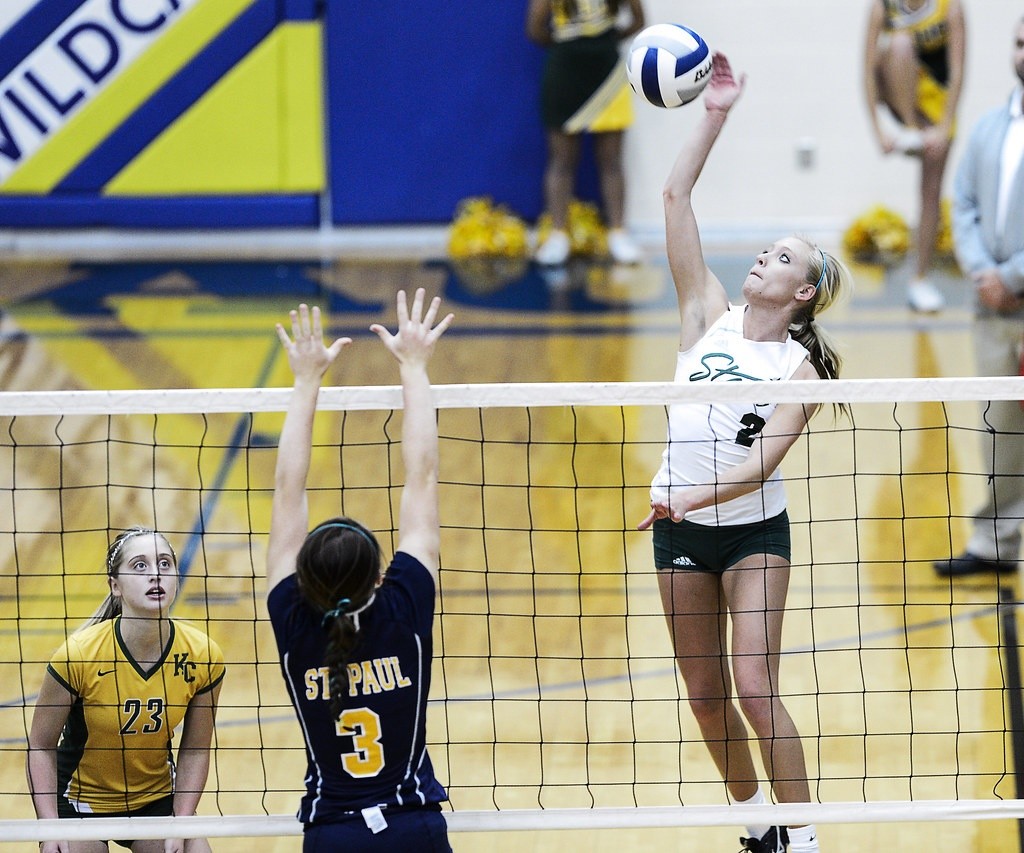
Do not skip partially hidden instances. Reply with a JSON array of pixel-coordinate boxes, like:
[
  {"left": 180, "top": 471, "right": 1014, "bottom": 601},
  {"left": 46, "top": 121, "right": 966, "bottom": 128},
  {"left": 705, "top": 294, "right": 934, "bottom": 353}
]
[{"left": 625, "top": 23, "right": 714, "bottom": 109}]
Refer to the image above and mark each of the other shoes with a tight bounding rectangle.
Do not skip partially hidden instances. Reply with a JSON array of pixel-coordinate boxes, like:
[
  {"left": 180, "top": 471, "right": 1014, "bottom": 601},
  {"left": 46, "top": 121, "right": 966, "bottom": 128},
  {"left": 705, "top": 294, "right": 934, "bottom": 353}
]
[{"left": 934, "top": 547, "right": 1018, "bottom": 578}]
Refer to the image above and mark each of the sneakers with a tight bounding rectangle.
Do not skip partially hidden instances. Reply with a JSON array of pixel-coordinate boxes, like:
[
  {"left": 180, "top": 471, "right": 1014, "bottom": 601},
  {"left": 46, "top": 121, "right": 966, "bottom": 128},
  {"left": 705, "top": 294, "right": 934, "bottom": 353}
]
[
  {"left": 739, "top": 826, "right": 783, "bottom": 853},
  {"left": 593, "top": 223, "right": 639, "bottom": 265},
  {"left": 532, "top": 227, "right": 575, "bottom": 267},
  {"left": 894, "top": 130, "right": 925, "bottom": 159},
  {"left": 908, "top": 279, "right": 939, "bottom": 313}
]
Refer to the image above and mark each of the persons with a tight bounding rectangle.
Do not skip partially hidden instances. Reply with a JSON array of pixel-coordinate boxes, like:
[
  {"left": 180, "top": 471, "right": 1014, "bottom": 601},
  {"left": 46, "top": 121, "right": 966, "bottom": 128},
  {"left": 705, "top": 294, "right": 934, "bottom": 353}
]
[
  {"left": 266, "top": 284, "right": 457, "bottom": 853},
  {"left": 932, "top": 12, "right": 1022, "bottom": 574},
  {"left": 521, "top": 1, "right": 647, "bottom": 270},
  {"left": 862, "top": 1, "right": 967, "bottom": 316},
  {"left": 633, "top": 52, "right": 855, "bottom": 853},
  {"left": 27, "top": 527, "right": 226, "bottom": 853}
]
